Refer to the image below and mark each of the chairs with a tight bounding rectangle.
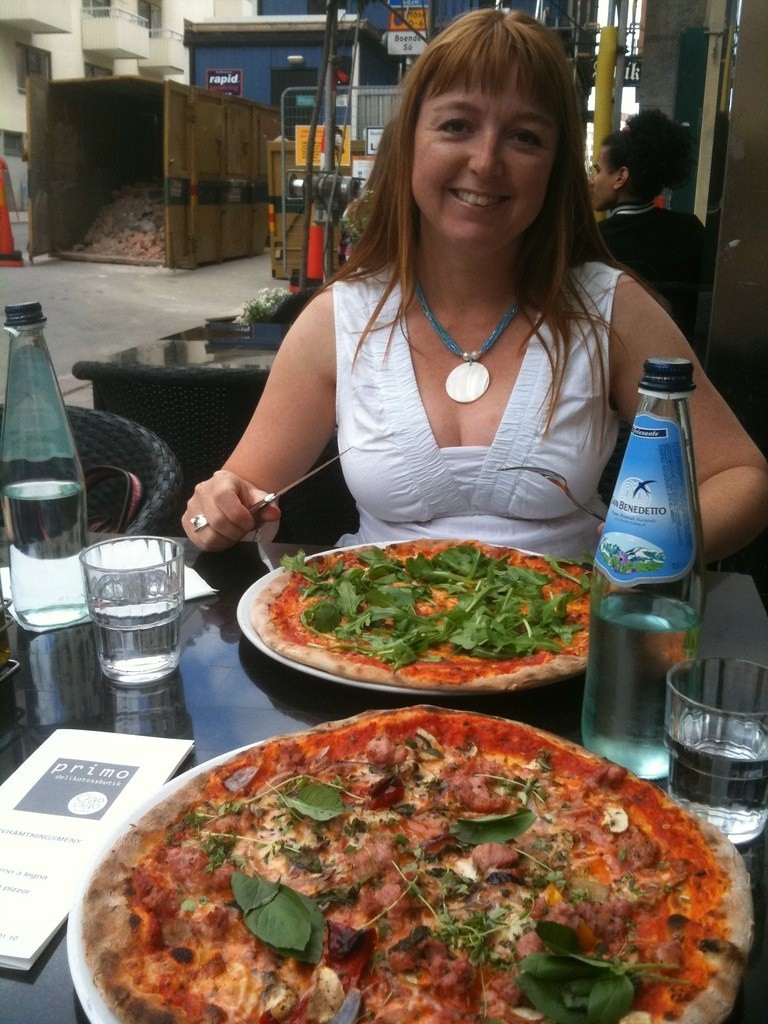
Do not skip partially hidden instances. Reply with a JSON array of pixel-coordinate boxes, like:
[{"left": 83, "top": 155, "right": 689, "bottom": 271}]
[
  {"left": 0, "top": 405, "right": 183, "bottom": 538},
  {"left": 71, "top": 362, "right": 270, "bottom": 487}
]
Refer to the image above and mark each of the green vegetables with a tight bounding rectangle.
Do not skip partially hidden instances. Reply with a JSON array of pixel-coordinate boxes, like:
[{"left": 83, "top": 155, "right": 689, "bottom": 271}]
[
  {"left": 277, "top": 517, "right": 594, "bottom": 675},
  {"left": 231, "top": 773, "right": 692, "bottom": 1024}
]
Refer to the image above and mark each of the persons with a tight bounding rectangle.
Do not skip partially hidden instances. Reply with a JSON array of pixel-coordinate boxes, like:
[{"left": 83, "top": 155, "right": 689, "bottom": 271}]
[
  {"left": 182, "top": 12, "right": 768, "bottom": 562},
  {"left": 588, "top": 108, "right": 713, "bottom": 368}
]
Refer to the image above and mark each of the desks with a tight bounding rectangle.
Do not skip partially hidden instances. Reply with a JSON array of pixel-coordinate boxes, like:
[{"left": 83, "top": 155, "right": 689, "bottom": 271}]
[{"left": 110, "top": 324, "right": 279, "bottom": 371}]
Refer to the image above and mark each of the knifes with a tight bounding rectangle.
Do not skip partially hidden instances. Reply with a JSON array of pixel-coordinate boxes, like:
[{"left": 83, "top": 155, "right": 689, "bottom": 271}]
[{"left": 248, "top": 445, "right": 359, "bottom": 516}]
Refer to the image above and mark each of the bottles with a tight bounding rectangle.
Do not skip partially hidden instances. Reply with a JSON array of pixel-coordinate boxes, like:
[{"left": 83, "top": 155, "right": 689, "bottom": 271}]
[
  {"left": 0, "top": 302, "right": 89, "bottom": 627},
  {"left": 580, "top": 357, "right": 700, "bottom": 783}
]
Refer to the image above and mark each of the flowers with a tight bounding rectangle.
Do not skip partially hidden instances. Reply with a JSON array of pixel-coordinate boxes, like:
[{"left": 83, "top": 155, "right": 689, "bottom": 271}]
[{"left": 233, "top": 288, "right": 293, "bottom": 324}]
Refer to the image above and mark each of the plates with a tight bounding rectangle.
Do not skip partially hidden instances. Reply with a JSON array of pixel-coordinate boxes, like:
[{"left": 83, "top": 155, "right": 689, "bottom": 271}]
[
  {"left": 237, "top": 537, "right": 541, "bottom": 695},
  {"left": 67, "top": 731, "right": 275, "bottom": 1024}
]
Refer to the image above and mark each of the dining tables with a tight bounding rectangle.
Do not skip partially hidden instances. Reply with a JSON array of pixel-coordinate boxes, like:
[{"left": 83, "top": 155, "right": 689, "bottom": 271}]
[{"left": 0, "top": 532, "right": 768, "bottom": 1024}]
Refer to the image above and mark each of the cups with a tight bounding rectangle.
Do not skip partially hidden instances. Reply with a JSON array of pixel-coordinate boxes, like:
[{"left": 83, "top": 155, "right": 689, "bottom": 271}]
[
  {"left": 79, "top": 537, "right": 181, "bottom": 684},
  {"left": 664, "top": 658, "right": 768, "bottom": 845}
]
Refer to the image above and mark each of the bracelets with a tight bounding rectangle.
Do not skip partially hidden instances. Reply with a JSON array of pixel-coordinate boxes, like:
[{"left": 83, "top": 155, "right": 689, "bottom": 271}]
[{"left": 253, "top": 528, "right": 260, "bottom": 542}]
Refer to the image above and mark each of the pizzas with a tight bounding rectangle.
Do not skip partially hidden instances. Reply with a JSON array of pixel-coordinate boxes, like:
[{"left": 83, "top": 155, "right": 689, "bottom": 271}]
[
  {"left": 252, "top": 538, "right": 593, "bottom": 691},
  {"left": 78, "top": 701, "right": 754, "bottom": 1024}
]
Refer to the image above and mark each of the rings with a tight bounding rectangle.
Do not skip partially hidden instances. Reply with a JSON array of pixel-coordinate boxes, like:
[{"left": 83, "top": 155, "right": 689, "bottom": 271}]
[{"left": 189, "top": 515, "right": 207, "bottom": 531}]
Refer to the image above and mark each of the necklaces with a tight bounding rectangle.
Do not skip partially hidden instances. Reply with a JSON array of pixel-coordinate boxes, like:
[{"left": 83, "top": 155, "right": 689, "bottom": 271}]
[{"left": 409, "top": 273, "right": 521, "bottom": 403}]
